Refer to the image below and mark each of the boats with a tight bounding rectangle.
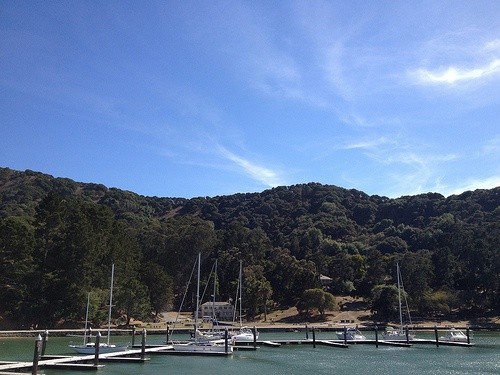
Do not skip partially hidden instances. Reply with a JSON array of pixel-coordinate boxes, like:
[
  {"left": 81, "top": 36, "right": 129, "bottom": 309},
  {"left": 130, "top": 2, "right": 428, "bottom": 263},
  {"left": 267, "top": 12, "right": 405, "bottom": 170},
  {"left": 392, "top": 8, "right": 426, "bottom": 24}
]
[
  {"left": 336, "top": 327, "right": 367, "bottom": 341},
  {"left": 438, "top": 329, "right": 472, "bottom": 342}
]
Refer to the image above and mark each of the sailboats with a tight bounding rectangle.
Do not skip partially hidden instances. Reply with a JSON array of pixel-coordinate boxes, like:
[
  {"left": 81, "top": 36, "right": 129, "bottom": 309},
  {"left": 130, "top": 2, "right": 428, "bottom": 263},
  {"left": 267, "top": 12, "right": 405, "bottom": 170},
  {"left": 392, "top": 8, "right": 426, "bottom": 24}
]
[
  {"left": 380, "top": 262, "right": 417, "bottom": 340},
  {"left": 165, "top": 252, "right": 260, "bottom": 354},
  {"left": 68, "top": 263, "right": 128, "bottom": 354}
]
